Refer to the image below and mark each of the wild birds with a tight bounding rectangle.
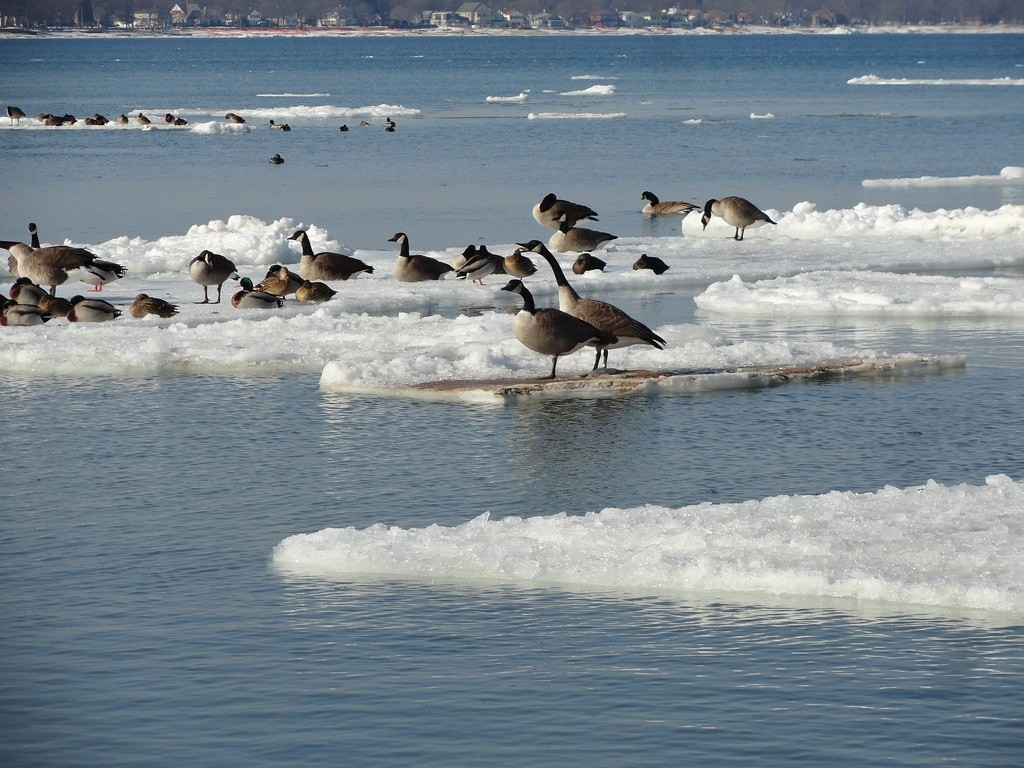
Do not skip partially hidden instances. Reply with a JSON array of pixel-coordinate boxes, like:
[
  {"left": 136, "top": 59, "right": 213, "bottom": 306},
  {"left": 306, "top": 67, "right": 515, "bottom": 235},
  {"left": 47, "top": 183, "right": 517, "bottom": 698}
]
[
  {"left": 189, "top": 230, "right": 374, "bottom": 309},
  {"left": 0, "top": 223, "right": 180, "bottom": 326},
  {"left": 387, "top": 191, "right": 777, "bottom": 380},
  {"left": 269, "top": 154, "right": 284, "bottom": 164},
  {"left": 6, "top": 106, "right": 396, "bottom": 132}
]
